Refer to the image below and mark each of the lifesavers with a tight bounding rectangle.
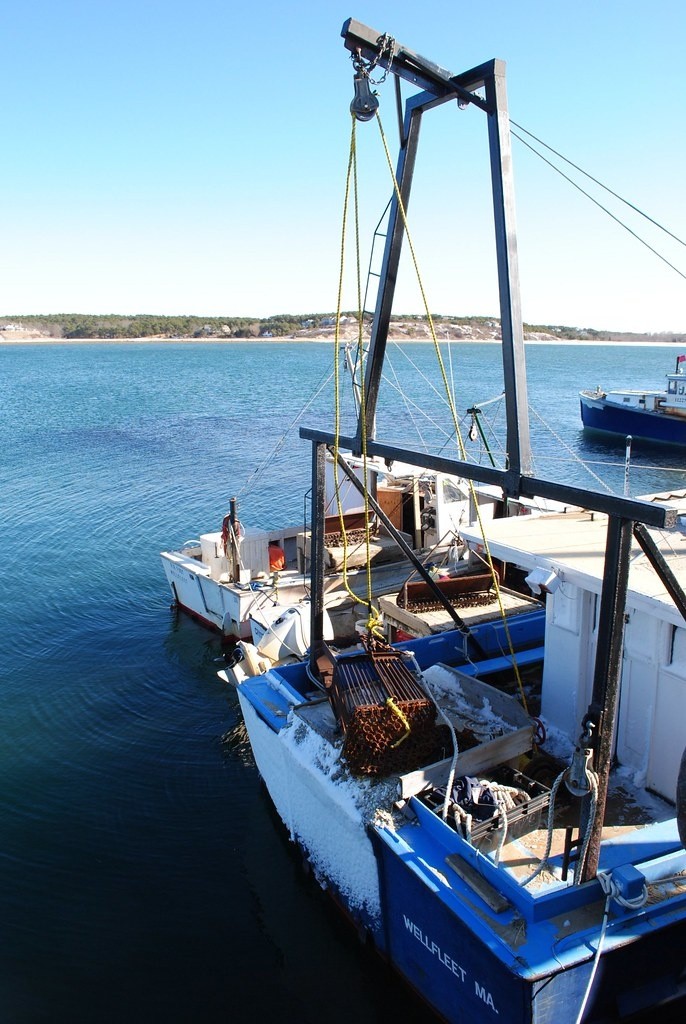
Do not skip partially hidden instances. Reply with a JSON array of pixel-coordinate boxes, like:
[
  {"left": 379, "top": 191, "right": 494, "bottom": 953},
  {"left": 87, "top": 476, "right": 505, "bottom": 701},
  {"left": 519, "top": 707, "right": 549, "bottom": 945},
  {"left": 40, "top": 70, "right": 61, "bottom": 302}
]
[{"left": 222, "top": 514, "right": 241, "bottom": 544}]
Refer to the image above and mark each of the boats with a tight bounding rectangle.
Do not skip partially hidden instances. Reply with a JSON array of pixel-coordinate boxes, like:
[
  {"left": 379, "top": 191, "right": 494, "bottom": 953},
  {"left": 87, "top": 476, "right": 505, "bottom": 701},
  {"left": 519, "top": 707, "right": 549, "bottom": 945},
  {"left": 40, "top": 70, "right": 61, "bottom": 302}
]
[
  {"left": 158, "top": 343, "right": 686, "bottom": 1023},
  {"left": 578, "top": 355, "right": 686, "bottom": 447}
]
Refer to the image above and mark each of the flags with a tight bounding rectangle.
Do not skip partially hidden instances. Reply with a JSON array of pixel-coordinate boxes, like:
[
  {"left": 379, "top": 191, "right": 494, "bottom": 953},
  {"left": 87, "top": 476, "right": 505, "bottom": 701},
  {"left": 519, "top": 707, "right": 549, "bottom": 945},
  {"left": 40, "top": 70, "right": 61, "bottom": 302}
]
[{"left": 678, "top": 356, "right": 685, "bottom": 362}]
